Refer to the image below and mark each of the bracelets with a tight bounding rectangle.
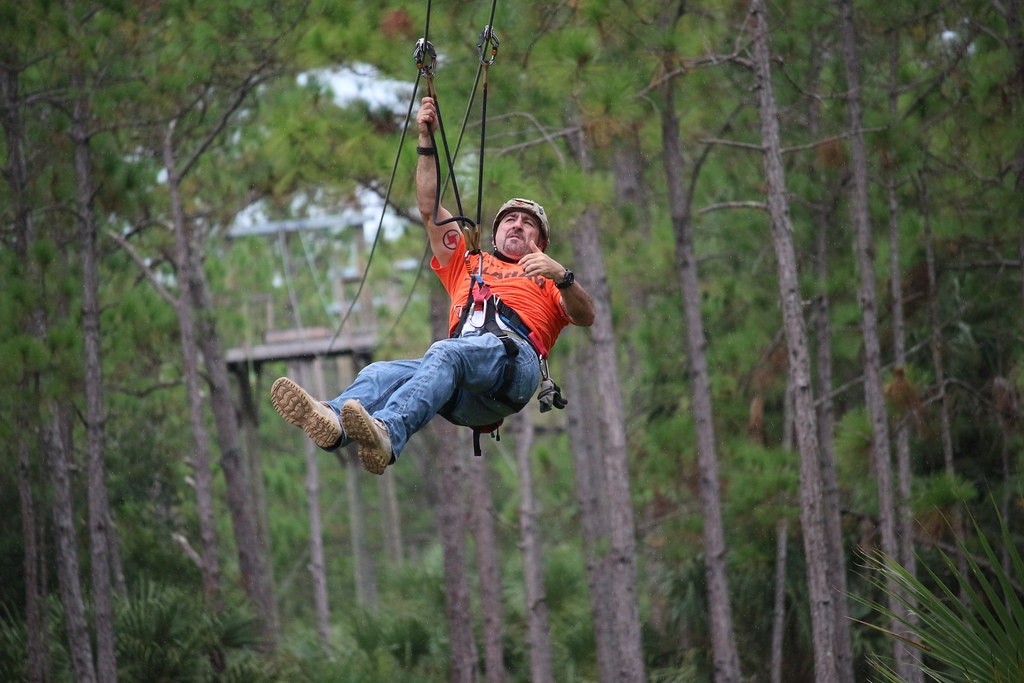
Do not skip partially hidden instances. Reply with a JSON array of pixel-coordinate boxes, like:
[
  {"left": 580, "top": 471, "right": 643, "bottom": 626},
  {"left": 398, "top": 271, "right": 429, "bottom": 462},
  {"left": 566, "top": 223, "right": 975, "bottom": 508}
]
[{"left": 416, "top": 145, "right": 435, "bottom": 155}]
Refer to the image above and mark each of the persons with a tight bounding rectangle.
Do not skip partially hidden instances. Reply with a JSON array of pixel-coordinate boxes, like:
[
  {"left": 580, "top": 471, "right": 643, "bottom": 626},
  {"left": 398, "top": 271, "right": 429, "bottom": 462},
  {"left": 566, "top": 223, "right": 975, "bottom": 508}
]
[{"left": 271, "top": 97, "right": 596, "bottom": 475}]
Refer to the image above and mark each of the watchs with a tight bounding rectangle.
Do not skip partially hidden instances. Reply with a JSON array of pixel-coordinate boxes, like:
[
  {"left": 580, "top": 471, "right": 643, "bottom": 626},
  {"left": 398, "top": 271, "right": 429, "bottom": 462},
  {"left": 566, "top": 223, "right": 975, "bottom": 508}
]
[{"left": 555, "top": 268, "right": 574, "bottom": 290}]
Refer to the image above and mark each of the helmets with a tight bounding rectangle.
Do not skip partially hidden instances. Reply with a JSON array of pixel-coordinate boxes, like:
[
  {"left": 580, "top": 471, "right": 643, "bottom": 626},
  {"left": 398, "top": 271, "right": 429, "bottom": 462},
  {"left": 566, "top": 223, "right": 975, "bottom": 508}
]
[{"left": 492, "top": 197, "right": 551, "bottom": 252}]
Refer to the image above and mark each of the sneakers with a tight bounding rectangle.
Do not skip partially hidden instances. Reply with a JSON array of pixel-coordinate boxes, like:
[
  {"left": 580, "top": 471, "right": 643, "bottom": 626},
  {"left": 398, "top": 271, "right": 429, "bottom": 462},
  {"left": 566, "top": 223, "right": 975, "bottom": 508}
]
[
  {"left": 339, "top": 399, "right": 394, "bottom": 475},
  {"left": 269, "top": 377, "right": 343, "bottom": 448}
]
